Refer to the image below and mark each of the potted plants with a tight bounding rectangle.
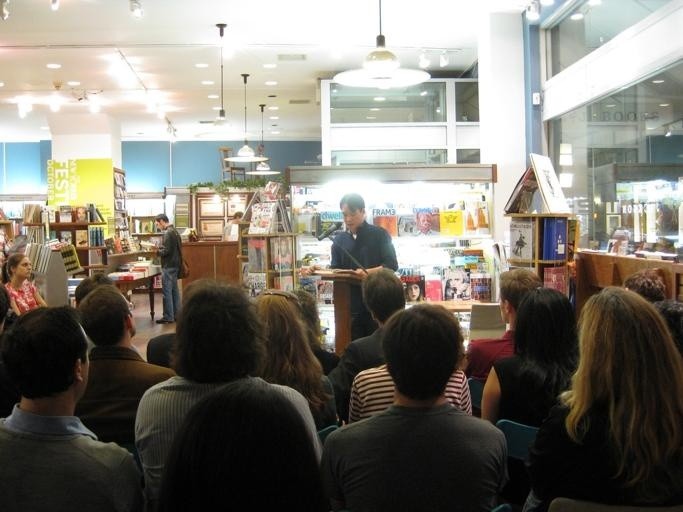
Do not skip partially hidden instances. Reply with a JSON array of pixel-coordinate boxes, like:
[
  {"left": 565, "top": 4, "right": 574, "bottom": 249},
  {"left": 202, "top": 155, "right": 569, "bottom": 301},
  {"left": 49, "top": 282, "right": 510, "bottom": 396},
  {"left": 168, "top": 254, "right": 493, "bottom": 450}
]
[{"left": 188, "top": 230, "right": 200, "bottom": 242}]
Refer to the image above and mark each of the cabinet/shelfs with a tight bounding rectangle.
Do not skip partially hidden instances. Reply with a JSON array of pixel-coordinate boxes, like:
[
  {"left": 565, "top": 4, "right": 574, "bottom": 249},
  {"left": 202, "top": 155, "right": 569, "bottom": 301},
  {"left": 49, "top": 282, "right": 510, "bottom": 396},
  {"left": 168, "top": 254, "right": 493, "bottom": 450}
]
[
  {"left": 287, "top": 163, "right": 498, "bottom": 341},
  {"left": 503, "top": 213, "right": 579, "bottom": 306},
  {"left": 1, "top": 215, "right": 166, "bottom": 320},
  {"left": 238, "top": 221, "right": 298, "bottom": 294}
]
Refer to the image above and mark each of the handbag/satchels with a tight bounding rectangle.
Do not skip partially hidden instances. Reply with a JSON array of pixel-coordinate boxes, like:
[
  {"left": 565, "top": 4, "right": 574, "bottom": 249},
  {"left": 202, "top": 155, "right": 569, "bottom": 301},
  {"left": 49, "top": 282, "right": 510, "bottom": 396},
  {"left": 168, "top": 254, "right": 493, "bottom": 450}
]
[{"left": 178, "top": 260, "right": 188, "bottom": 279}]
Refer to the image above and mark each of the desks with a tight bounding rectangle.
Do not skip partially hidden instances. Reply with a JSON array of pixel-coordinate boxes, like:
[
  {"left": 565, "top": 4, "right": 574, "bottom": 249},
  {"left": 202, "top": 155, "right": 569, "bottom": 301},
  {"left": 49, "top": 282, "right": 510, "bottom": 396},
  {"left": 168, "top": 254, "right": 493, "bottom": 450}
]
[{"left": 577, "top": 249, "right": 683, "bottom": 305}]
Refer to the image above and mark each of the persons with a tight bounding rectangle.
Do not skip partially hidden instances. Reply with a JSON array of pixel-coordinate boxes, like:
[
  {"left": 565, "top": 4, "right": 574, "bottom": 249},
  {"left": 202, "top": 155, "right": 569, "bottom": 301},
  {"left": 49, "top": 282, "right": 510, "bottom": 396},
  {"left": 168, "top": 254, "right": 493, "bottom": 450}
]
[
  {"left": 222, "top": 211, "right": 244, "bottom": 241},
  {"left": 406, "top": 282, "right": 423, "bottom": 302},
  {"left": 332, "top": 193, "right": 400, "bottom": 344},
  {"left": 0, "top": 251, "right": 683, "bottom": 512},
  {"left": 152, "top": 215, "right": 181, "bottom": 323}
]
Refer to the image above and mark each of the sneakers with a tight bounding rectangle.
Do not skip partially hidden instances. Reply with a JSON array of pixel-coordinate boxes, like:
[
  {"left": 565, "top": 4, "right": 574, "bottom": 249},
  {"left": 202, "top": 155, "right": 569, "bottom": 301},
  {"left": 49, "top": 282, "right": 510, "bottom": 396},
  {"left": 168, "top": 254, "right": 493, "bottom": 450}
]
[{"left": 155, "top": 319, "right": 169, "bottom": 324}]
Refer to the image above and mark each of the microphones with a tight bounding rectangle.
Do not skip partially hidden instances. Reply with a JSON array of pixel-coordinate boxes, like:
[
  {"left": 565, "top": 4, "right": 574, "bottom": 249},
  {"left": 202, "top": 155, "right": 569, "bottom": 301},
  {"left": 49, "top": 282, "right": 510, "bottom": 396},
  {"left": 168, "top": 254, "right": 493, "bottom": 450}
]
[{"left": 318, "top": 223, "right": 341, "bottom": 241}]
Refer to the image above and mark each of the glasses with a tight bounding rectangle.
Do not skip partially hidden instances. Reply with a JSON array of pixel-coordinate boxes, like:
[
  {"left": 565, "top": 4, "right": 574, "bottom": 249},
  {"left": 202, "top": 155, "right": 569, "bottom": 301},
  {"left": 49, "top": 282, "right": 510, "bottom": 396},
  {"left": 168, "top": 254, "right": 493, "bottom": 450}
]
[{"left": 126, "top": 300, "right": 134, "bottom": 310}]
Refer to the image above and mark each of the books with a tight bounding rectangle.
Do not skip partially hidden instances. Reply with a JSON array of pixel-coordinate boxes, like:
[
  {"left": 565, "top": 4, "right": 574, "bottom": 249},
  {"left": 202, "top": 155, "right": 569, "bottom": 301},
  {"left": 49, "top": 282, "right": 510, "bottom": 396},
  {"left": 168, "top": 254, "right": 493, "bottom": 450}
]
[
  {"left": 504, "top": 152, "right": 579, "bottom": 299},
  {"left": 371, "top": 191, "right": 496, "bottom": 349},
  {"left": 401, "top": 276, "right": 425, "bottom": 302},
  {"left": 0, "top": 168, "right": 168, "bottom": 306},
  {"left": 242, "top": 182, "right": 345, "bottom": 304},
  {"left": 441, "top": 265, "right": 469, "bottom": 300},
  {"left": 314, "top": 268, "right": 359, "bottom": 279},
  {"left": 466, "top": 262, "right": 493, "bottom": 301}
]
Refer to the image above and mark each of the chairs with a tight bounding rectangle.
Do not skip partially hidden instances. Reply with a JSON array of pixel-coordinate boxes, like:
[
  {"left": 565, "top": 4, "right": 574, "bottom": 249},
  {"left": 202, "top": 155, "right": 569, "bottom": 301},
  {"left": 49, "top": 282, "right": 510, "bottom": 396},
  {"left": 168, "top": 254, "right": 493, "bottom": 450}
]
[{"left": 217, "top": 146, "right": 246, "bottom": 182}]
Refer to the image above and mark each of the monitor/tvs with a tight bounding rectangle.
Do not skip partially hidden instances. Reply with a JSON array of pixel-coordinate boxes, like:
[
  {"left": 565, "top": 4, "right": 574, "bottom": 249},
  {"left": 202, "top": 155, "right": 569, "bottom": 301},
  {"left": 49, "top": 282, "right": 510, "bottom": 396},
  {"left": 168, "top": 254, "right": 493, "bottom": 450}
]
[{"left": 188, "top": 230, "right": 198, "bottom": 242}]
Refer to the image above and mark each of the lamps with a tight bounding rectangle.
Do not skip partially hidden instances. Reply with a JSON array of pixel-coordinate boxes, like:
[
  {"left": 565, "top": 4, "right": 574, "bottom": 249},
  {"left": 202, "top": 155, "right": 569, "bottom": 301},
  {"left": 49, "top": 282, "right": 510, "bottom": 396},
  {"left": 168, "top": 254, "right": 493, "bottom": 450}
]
[
  {"left": 526, "top": 0, "right": 541, "bottom": 21},
  {"left": 231, "top": 0, "right": 281, "bottom": 177},
  {"left": 570, "top": 8, "right": 583, "bottom": 19},
  {"left": 333, "top": 1, "right": 431, "bottom": 88}
]
